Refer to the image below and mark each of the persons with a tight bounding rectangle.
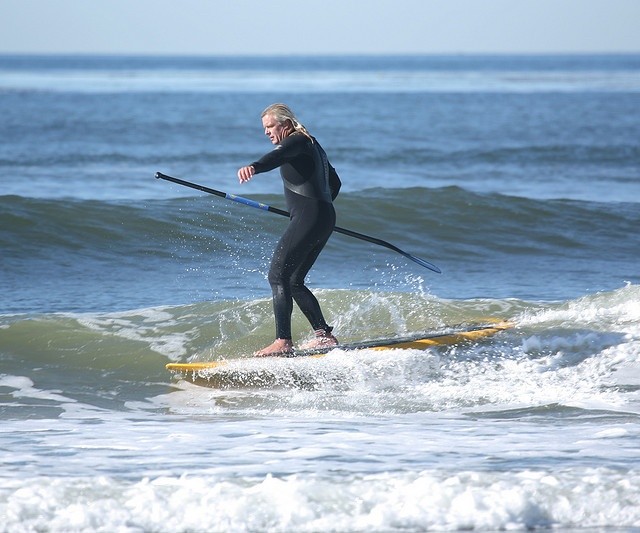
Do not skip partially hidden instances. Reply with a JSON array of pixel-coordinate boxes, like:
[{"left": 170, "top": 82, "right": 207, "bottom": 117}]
[{"left": 238, "top": 105, "right": 342, "bottom": 357}]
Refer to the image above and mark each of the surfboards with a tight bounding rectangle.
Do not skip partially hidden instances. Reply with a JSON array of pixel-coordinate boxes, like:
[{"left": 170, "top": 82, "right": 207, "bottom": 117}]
[{"left": 166, "top": 318, "right": 513, "bottom": 372}]
